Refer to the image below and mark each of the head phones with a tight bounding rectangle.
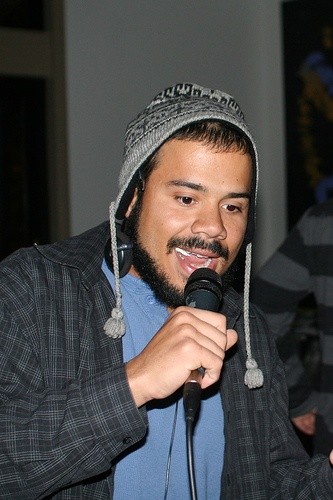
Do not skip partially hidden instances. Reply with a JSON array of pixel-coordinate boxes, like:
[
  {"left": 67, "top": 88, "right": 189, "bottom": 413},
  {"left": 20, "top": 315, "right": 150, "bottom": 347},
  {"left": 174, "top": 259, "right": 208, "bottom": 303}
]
[{"left": 103, "top": 223, "right": 239, "bottom": 293}]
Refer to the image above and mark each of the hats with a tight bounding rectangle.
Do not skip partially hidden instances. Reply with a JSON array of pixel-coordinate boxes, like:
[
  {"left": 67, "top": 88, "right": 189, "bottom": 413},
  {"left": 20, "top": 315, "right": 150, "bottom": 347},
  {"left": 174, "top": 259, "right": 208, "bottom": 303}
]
[{"left": 102, "top": 81, "right": 264, "bottom": 389}]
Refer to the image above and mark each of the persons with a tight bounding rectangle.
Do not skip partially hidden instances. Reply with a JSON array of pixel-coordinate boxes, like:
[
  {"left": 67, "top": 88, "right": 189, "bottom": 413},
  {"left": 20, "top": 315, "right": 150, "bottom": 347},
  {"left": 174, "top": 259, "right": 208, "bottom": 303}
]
[
  {"left": 248, "top": 24, "right": 333, "bottom": 458},
  {"left": 0, "top": 84, "right": 333, "bottom": 500}
]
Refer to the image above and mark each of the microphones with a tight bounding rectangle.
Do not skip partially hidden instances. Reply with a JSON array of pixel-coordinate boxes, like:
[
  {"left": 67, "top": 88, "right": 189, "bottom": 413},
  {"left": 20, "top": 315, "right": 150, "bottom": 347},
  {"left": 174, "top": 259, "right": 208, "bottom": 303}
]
[{"left": 183, "top": 267, "right": 224, "bottom": 420}]
